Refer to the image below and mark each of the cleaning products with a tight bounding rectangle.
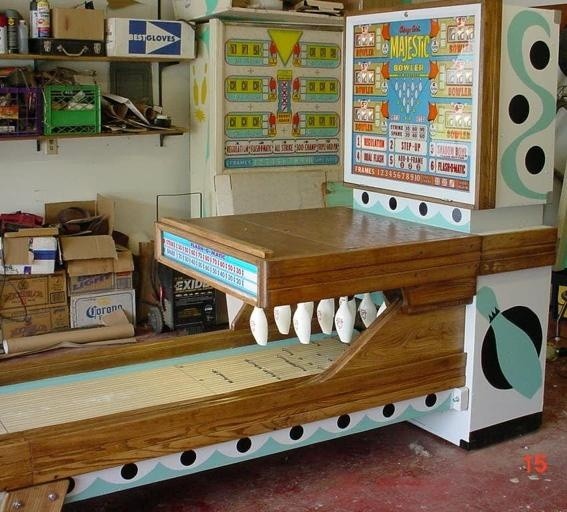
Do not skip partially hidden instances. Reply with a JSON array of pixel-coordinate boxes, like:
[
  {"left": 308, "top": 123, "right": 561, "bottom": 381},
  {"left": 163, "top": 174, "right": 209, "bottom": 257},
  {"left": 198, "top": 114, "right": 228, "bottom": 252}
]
[{"left": 17, "top": 19, "right": 28, "bottom": 54}]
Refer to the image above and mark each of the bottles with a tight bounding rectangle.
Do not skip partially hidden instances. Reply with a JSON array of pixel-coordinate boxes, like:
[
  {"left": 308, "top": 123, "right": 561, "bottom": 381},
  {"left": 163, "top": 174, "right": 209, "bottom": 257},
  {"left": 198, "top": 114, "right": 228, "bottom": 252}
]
[{"left": 0, "top": 0, "right": 51, "bottom": 54}]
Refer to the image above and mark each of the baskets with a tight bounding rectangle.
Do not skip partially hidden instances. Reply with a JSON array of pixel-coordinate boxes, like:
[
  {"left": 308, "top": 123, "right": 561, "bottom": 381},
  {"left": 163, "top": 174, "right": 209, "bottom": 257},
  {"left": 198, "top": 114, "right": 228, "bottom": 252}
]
[{"left": 0, "top": 84, "right": 102, "bottom": 139}]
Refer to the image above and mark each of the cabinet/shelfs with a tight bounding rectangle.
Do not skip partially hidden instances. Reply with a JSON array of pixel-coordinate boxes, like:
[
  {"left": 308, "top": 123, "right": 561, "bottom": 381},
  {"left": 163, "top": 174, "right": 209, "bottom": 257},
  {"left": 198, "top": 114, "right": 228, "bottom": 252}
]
[{"left": 0, "top": 53, "right": 188, "bottom": 141}]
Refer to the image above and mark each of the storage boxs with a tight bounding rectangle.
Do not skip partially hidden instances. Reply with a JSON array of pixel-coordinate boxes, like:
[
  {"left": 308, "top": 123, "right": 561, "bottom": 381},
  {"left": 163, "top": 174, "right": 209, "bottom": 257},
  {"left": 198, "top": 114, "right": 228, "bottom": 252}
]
[
  {"left": 50, "top": 7, "right": 105, "bottom": 43},
  {"left": 106, "top": 17, "right": 196, "bottom": 56},
  {"left": 0, "top": 193, "right": 135, "bottom": 344}
]
